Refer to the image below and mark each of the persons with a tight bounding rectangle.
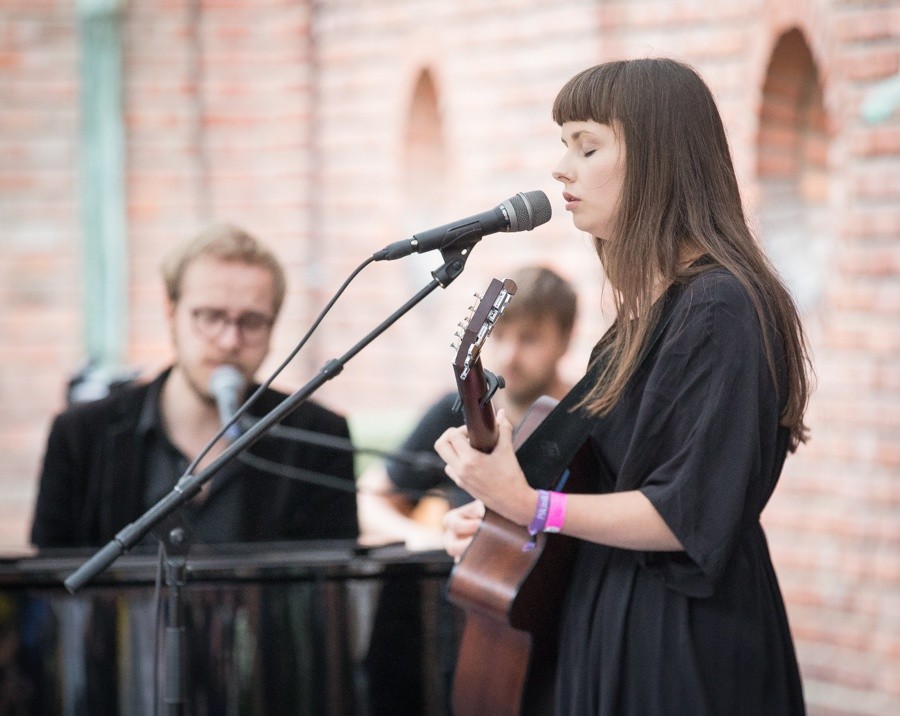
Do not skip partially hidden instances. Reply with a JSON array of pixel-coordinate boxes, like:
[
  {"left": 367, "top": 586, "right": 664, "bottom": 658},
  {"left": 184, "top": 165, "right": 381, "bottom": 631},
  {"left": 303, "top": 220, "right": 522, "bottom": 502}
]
[
  {"left": 356, "top": 265, "right": 573, "bottom": 550},
  {"left": 27, "top": 222, "right": 366, "bottom": 559},
  {"left": 432, "top": 57, "right": 815, "bottom": 716}
]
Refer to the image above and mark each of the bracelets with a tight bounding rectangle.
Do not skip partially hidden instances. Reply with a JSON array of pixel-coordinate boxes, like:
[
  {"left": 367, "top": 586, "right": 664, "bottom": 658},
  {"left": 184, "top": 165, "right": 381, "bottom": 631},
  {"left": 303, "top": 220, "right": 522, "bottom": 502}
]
[{"left": 523, "top": 490, "right": 568, "bottom": 552}]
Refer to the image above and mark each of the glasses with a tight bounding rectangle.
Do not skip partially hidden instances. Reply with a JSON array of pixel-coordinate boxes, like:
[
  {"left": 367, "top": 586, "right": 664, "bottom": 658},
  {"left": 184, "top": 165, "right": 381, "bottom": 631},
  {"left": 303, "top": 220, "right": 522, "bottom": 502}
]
[{"left": 175, "top": 299, "right": 274, "bottom": 339}]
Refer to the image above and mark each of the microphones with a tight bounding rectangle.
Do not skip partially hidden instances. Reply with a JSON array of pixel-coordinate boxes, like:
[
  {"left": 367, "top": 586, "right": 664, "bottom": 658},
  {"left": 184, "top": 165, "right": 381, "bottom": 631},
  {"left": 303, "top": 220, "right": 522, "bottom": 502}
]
[
  {"left": 373, "top": 190, "right": 552, "bottom": 264},
  {"left": 206, "top": 362, "right": 249, "bottom": 450}
]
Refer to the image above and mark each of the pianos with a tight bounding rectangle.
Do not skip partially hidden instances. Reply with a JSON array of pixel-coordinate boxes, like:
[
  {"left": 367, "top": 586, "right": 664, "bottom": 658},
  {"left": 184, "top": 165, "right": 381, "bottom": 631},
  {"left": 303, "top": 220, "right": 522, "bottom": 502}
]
[{"left": 0, "top": 534, "right": 459, "bottom": 716}]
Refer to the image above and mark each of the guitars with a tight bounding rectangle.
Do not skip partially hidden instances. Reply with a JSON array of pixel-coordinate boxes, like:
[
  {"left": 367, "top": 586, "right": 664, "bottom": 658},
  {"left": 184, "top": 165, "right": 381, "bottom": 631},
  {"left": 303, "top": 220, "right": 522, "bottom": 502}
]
[{"left": 446, "top": 276, "right": 617, "bottom": 716}]
